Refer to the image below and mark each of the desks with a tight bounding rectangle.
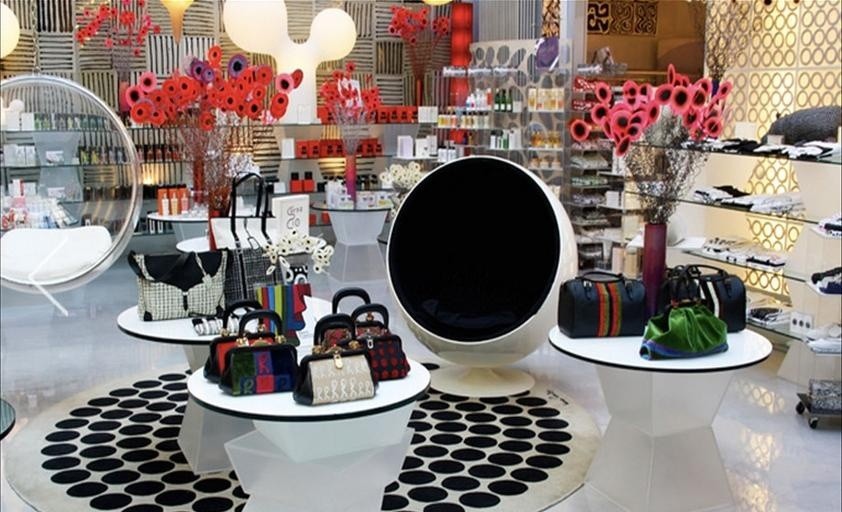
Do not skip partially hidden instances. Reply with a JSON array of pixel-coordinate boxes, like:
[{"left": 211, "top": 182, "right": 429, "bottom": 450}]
[
  {"left": 546, "top": 321, "right": 772, "bottom": 512},
  {"left": 176, "top": 232, "right": 330, "bottom": 293},
  {"left": 146, "top": 206, "right": 277, "bottom": 244},
  {"left": 117, "top": 295, "right": 339, "bottom": 476},
  {"left": 305, "top": 196, "right": 403, "bottom": 282}
]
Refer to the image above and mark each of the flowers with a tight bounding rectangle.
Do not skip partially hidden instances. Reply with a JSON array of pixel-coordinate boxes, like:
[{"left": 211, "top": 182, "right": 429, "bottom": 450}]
[
  {"left": 74, "top": 0, "right": 161, "bottom": 82},
  {"left": 387, "top": 7, "right": 448, "bottom": 78},
  {"left": 320, "top": 61, "right": 380, "bottom": 155},
  {"left": 571, "top": 64, "right": 732, "bottom": 226}
]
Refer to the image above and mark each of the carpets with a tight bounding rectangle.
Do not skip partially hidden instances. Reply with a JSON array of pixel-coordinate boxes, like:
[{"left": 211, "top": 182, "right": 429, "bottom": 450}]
[{"left": 0, "top": 365, "right": 605, "bottom": 512}]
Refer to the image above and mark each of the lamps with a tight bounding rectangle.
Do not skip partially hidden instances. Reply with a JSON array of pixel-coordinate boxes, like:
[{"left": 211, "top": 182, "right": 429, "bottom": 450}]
[{"left": 0, "top": 3, "right": 20, "bottom": 61}]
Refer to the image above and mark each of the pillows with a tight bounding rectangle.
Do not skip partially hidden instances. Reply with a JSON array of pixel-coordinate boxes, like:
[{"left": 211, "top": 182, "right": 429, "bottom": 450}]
[
  {"left": 423, "top": 296, "right": 517, "bottom": 333},
  {"left": 760, "top": 105, "right": 842, "bottom": 144},
  {"left": 0, "top": 226, "right": 113, "bottom": 284}
]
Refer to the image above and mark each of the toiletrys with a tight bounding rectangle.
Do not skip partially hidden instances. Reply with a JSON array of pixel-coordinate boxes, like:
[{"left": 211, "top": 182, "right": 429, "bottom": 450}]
[
  {"left": 301, "top": 111, "right": 418, "bottom": 158},
  {"left": 35, "top": 117, "right": 189, "bottom": 217},
  {"left": 436, "top": 87, "right": 513, "bottom": 169}
]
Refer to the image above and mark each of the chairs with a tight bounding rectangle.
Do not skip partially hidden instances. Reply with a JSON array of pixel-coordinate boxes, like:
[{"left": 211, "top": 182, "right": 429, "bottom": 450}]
[
  {"left": 385, "top": 155, "right": 579, "bottom": 398},
  {"left": 1, "top": 77, "right": 142, "bottom": 314}
]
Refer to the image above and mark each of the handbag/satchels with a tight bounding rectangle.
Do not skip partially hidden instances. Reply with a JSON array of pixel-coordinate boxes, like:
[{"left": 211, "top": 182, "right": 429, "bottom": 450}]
[
  {"left": 659, "top": 272, "right": 746, "bottom": 333},
  {"left": 638, "top": 265, "right": 728, "bottom": 361},
  {"left": 208, "top": 173, "right": 279, "bottom": 250},
  {"left": 224, "top": 245, "right": 284, "bottom": 305},
  {"left": 204, "top": 283, "right": 410, "bottom": 407},
  {"left": 556, "top": 274, "right": 646, "bottom": 338},
  {"left": 130, "top": 249, "right": 225, "bottom": 321}
]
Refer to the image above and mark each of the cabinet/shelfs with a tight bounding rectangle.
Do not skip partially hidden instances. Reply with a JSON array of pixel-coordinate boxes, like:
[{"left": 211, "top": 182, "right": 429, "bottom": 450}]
[
  {"left": 0, "top": 131, "right": 196, "bottom": 236},
  {"left": 190, "top": 360, "right": 435, "bottom": 511},
  {"left": 594, "top": 143, "right": 842, "bottom": 355},
  {"left": 386, "top": 66, "right": 565, "bottom": 203}
]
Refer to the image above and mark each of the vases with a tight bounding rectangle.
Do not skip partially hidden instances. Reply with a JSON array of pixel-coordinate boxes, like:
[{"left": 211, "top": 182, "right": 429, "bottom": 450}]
[
  {"left": 344, "top": 155, "right": 357, "bottom": 204},
  {"left": 119, "top": 68, "right": 131, "bottom": 111},
  {"left": 643, "top": 222, "right": 668, "bottom": 322},
  {"left": 189, "top": 161, "right": 205, "bottom": 207},
  {"left": 415, "top": 79, "right": 423, "bottom": 114},
  {"left": 207, "top": 206, "right": 221, "bottom": 252}
]
